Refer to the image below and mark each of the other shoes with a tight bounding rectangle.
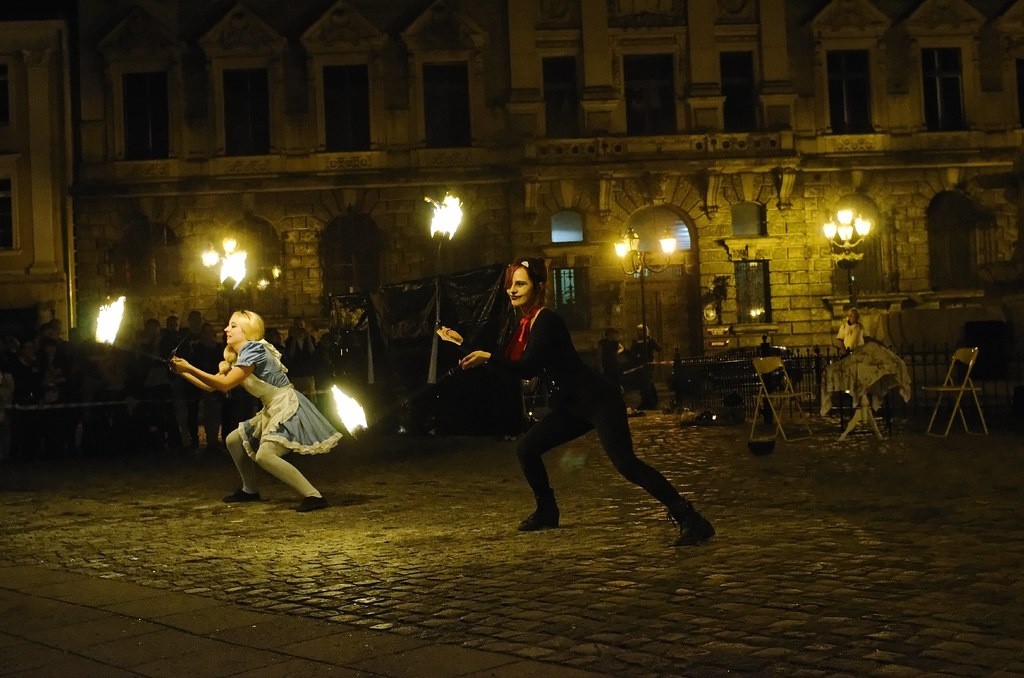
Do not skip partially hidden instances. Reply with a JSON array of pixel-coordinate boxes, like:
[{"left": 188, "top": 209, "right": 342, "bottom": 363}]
[
  {"left": 223, "top": 489, "right": 260, "bottom": 502},
  {"left": 297, "top": 495, "right": 327, "bottom": 512}
]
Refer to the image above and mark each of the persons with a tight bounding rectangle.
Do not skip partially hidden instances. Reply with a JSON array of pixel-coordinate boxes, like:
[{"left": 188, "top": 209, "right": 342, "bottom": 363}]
[
  {"left": 170, "top": 310, "right": 344, "bottom": 512},
  {"left": 836, "top": 308, "right": 864, "bottom": 354},
  {"left": 436, "top": 257, "right": 715, "bottom": 547},
  {"left": 597, "top": 327, "right": 624, "bottom": 379},
  {"left": 0, "top": 311, "right": 253, "bottom": 457},
  {"left": 631, "top": 324, "right": 660, "bottom": 409},
  {"left": 273, "top": 316, "right": 333, "bottom": 403}
]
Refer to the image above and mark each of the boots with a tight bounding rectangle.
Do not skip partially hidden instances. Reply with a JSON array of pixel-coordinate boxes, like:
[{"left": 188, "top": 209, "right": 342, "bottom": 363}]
[
  {"left": 667, "top": 499, "right": 715, "bottom": 547},
  {"left": 518, "top": 488, "right": 559, "bottom": 530}
]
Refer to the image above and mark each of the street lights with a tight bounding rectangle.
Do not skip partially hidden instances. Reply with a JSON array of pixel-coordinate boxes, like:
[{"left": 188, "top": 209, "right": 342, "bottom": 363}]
[
  {"left": 824, "top": 210, "right": 873, "bottom": 309},
  {"left": 203, "top": 236, "right": 248, "bottom": 320},
  {"left": 614, "top": 226, "right": 680, "bottom": 361}
]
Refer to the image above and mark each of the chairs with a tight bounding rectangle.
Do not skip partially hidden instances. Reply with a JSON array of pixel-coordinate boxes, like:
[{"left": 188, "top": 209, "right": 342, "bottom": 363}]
[
  {"left": 921, "top": 346, "right": 988, "bottom": 438},
  {"left": 748, "top": 354, "right": 814, "bottom": 443}
]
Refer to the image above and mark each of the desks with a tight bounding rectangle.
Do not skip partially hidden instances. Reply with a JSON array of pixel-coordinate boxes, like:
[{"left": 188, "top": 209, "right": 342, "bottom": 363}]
[{"left": 821, "top": 341, "right": 911, "bottom": 442}]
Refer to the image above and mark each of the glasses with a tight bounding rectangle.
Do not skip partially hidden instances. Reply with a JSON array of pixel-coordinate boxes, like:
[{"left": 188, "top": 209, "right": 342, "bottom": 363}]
[{"left": 514, "top": 257, "right": 535, "bottom": 275}]
